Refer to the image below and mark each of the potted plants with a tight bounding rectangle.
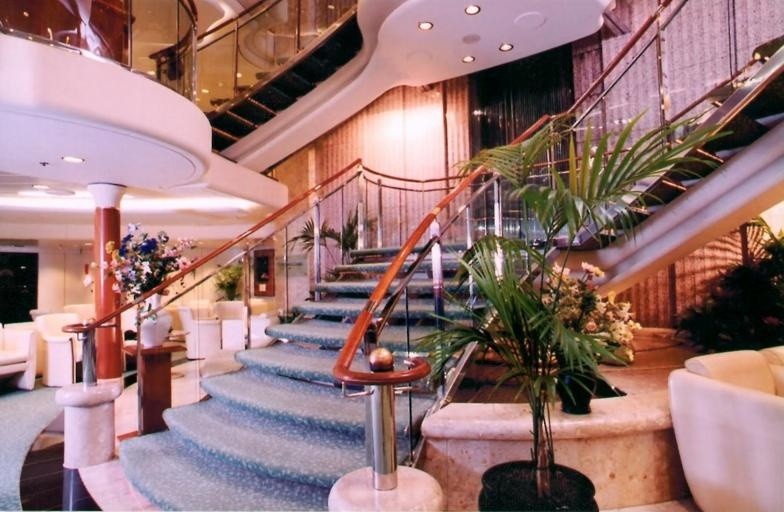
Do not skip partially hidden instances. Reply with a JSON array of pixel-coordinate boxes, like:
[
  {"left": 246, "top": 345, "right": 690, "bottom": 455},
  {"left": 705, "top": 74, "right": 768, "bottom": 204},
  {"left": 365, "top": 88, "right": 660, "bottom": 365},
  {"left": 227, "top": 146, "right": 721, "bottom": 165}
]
[{"left": 409, "top": 109, "right": 735, "bottom": 511}]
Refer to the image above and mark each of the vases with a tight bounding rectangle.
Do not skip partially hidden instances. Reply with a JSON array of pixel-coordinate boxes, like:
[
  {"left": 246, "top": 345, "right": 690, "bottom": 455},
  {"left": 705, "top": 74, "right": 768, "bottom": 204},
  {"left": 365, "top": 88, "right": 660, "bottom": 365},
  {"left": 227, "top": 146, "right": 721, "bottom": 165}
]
[
  {"left": 558, "top": 363, "right": 597, "bottom": 414},
  {"left": 132, "top": 293, "right": 173, "bottom": 347}
]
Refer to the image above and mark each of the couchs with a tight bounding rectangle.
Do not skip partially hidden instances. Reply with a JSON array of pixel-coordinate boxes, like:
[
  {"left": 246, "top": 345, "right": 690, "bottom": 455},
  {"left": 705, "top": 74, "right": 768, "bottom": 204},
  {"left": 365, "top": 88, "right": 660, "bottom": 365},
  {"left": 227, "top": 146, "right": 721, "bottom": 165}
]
[{"left": 667, "top": 344, "right": 784, "bottom": 511}]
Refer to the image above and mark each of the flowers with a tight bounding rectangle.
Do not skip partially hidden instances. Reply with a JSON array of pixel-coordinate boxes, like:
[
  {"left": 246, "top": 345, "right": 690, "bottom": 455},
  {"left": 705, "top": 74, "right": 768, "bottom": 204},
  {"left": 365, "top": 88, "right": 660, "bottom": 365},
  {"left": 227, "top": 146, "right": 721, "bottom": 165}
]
[
  {"left": 542, "top": 261, "right": 643, "bottom": 363},
  {"left": 83, "top": 222, "right": 205, "bottom": 327}
]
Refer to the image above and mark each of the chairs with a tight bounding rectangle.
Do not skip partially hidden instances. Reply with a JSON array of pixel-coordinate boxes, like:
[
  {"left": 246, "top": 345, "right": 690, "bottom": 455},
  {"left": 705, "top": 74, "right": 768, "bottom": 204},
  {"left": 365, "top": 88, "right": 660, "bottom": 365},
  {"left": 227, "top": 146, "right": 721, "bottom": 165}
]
[{"left": 0, "top": 301, "right": 281, "bottom": 391}]
